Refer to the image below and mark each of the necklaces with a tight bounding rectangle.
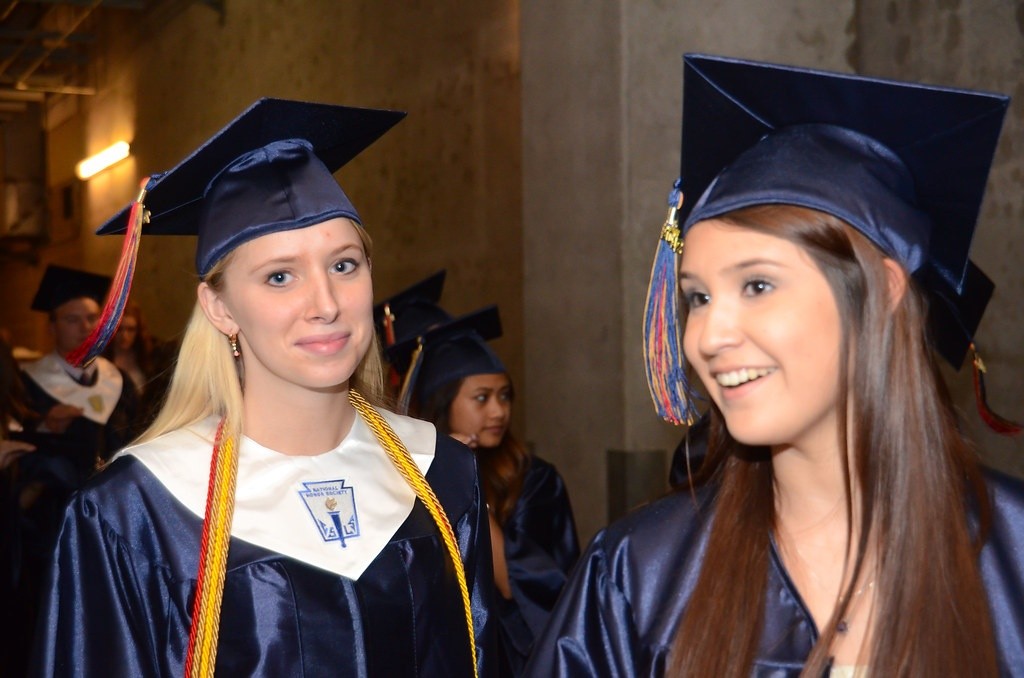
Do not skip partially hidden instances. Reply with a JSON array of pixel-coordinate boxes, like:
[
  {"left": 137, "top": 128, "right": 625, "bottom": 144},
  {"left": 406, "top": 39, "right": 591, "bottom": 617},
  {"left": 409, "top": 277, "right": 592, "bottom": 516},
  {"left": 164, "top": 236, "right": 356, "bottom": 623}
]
[{"left": 794, "top": 546, "right": 884, "bottom": 635}]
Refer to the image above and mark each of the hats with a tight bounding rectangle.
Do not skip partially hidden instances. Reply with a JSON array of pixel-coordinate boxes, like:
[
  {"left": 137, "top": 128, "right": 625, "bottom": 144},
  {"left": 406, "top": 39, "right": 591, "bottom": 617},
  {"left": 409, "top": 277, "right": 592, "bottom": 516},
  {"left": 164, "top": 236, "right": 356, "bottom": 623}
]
[
  {"left": 28, "top": 263, "right": 115, "bottom": 320},
  {"left": 67, "top": 95, "right": 411, "bottom": 369},
  {"left": 643, "top": 52, "right": 1007, "bottom": 425},
  {"left": 380, "top": 302, "right": 507, "bottom": 417},
  {"left": 373, "top": 267, "right": 448, "bottom": 371}
]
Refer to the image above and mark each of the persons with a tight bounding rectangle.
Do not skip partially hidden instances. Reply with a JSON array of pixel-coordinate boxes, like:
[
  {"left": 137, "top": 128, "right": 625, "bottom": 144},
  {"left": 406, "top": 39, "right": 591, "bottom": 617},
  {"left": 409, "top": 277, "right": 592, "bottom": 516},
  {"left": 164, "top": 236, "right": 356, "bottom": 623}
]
[
  {"left": 0, "top": 262, "right": 162, "bottom": 677},
  {"left": 42, "top": 98, "right": 504, "bottom": 675},
  {"left": 377, "top": 269, "right": 581, "bottom": 675},
  {"left": 527, "top": 50, "right": 1022, "bottom": 677}
]
